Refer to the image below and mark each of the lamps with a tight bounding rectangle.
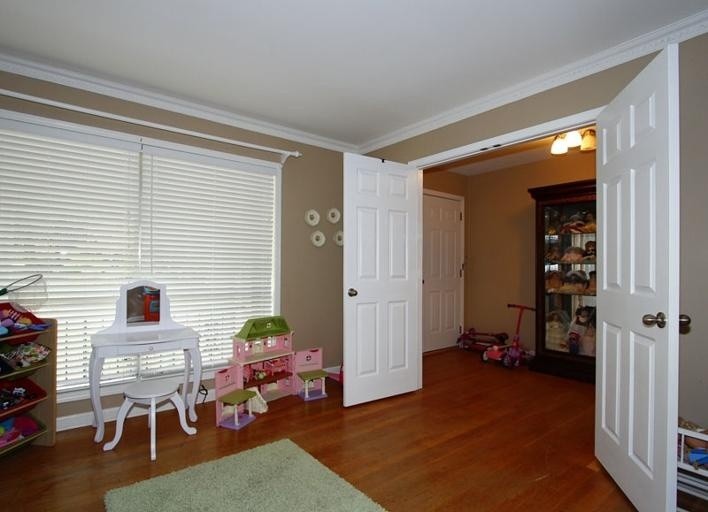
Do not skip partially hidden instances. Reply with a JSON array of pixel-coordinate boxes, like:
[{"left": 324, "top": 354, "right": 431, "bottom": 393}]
[{"left": 549, "top": 128, "right": 597, "bottom": 155}]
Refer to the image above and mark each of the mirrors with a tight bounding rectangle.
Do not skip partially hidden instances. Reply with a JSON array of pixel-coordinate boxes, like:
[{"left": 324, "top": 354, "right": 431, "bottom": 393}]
[{"left": 117, "top": 282, "right": 171, "bottom": 328}]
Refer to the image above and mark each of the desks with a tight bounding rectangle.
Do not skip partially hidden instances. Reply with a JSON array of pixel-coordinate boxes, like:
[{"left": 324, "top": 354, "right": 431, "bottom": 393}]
[{"left": 86, "top": 327, "right": 201, "bottom": 444}]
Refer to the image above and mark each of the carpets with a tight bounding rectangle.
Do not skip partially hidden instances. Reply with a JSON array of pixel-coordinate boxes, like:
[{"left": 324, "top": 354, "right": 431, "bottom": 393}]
[{"left": 103, "top": 437, "right": 386, "bottom": 512}]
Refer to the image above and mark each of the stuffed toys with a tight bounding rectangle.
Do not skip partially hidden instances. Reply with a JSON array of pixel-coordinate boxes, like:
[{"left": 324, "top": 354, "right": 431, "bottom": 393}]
[{"left": 545, "top": 206, "right": 596, "bottom": 360}]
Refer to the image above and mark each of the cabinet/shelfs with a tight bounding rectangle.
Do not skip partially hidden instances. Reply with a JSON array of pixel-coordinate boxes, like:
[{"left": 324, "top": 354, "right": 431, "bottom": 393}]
[
  {"left": 0, "top": 301, "right": 58, "bottom": 456},
  {"left": 526, "top": 177, "right": 597, "bottom": 381}
]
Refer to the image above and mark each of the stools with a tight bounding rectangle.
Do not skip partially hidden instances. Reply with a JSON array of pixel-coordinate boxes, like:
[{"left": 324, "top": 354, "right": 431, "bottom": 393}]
[{"left": 101, "top": 377, "right": 197, "bottom": 462}]
